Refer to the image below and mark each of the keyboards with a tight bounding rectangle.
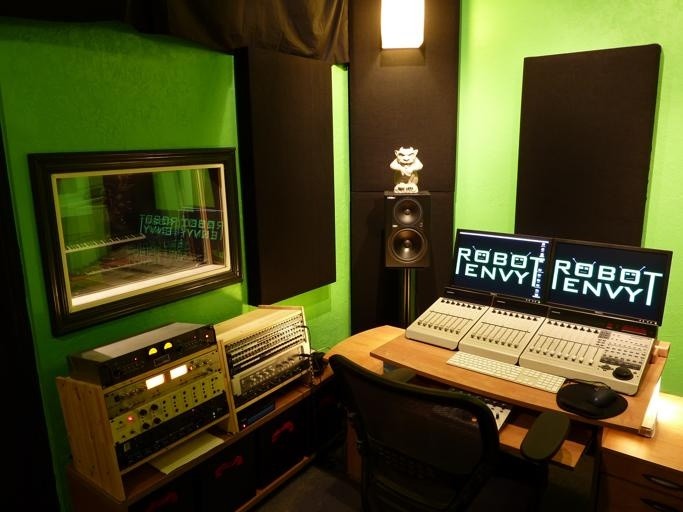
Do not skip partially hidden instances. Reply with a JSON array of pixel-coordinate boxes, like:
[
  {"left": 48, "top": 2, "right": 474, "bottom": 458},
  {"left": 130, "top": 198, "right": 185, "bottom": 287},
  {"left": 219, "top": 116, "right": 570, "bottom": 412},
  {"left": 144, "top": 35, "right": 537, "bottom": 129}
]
[{"left": 446, "top": 351, "right": 565, "bottom": 393}]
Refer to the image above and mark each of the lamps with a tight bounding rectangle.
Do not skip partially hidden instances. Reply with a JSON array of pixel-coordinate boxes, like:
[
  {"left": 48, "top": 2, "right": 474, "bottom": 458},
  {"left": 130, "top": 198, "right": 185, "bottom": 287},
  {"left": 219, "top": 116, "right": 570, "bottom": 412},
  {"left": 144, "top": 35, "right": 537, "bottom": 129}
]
[{"left": 379, "top": 0, "right": 426, "bottom": 68}]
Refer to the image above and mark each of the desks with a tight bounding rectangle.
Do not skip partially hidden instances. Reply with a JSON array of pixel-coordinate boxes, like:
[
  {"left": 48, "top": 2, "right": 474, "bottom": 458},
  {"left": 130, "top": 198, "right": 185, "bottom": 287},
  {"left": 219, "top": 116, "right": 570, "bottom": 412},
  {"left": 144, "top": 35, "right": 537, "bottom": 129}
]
[{"left": 323, "top": 325, "right": 671, "bottom": 512}]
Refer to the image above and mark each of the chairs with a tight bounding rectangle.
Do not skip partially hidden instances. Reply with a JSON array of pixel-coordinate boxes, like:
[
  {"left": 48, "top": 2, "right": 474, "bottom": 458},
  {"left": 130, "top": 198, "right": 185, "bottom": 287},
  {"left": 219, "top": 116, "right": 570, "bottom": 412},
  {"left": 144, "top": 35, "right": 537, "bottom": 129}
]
[{"left": 326, "top": 355, "right": 572, "bottom": 512}]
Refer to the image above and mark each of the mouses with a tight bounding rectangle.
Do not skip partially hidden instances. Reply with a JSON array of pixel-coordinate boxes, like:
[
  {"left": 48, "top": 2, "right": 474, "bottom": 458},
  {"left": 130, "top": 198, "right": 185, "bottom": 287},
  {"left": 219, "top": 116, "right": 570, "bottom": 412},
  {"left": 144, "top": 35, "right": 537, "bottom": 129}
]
[{"left": 590, "top": 392, "right": 617, "bottom": 406}]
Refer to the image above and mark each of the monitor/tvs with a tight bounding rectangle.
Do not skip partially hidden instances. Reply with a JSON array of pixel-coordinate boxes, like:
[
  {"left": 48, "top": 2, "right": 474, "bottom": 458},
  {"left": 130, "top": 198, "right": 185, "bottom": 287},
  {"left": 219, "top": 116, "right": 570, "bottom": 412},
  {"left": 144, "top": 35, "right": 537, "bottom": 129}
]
[
  {"left": 449, "top": 228, "right": 553, "bottom": 305},
  {"left": 546, "top": 238, "right": 673, "bottom": 327}
]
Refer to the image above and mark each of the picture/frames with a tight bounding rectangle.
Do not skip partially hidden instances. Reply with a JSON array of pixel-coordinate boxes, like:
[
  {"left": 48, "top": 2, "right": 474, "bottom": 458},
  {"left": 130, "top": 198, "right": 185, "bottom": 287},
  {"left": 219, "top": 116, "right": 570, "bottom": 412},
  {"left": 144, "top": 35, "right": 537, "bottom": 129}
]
[{"left": 28, "top": 147, "right": 243, "bottom": 337}]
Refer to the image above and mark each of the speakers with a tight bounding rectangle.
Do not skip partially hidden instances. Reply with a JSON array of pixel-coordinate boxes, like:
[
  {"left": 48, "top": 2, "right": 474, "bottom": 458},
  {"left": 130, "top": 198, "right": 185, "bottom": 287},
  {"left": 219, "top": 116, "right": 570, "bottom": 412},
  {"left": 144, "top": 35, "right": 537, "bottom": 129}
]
[{"left": 384, "top": 190, "right": 433, "bottom": 269}]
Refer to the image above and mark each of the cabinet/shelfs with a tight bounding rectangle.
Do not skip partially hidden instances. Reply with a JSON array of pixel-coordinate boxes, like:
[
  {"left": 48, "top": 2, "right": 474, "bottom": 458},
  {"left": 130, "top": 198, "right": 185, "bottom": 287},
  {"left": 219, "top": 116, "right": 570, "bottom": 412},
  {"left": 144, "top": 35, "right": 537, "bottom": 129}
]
[
  {"left": 597, "top": 392, "right": 683, "bottom": 512},
  {"left": 63, "top": 362, "right": 339, "bottom": 512}
]
